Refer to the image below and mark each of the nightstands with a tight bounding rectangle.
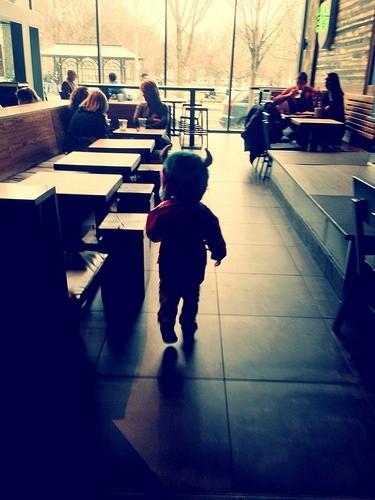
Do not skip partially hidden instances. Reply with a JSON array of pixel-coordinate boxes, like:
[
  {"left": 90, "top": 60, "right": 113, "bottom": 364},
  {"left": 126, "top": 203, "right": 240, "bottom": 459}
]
[{"left": 137, "top": 164, "right": 164, "bottom": 207}]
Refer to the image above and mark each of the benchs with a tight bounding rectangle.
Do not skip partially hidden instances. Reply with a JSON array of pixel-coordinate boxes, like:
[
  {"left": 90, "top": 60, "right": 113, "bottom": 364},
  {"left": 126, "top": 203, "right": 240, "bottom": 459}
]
[
  {"left": 271, "top": 92, "right": 375, "bottom": 152},
  {"left": 0, "top": 99, "right": 147, "bottom": 181},
  {"left": 0, "top": 182, "right": 110, "bottom": 362}
]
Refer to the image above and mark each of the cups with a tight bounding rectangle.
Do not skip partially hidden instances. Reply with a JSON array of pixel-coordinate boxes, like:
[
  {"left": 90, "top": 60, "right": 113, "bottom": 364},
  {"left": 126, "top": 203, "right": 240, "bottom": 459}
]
[
  {"left": 118, "top": 119, "right": 128, "bottom": 132},
  {"left": 139, "top": 117, "right": 147, "bottom": 132},
  {"left": 314, "top": 108, "right": 322, "bottom": 117},
  {"left": 296, "top": 89, "right": 301, "bottom": 98},
  {"left": 117, "top": 94, "right": 123, "bottom": 102}
]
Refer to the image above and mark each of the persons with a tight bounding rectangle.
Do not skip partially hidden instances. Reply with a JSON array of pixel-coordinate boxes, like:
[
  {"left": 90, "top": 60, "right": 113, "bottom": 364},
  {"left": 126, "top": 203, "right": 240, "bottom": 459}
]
[
  {"left": 68, "top": 86, "right": 91, "bottom": 113},
  {"left": 64, "top": 89, "right": 114, "bottom": 154},
  {"left": 314, "top": 72, "right": 346, "bottom": 140},
  {"left": 134, "top": 78, "right": 171, "bottom": 154},
  {"left": 61, "top": 69, "right": 78, "bottom": 98},
  {"left": 272, "top": 72, "right": 317, "bottom": 113},
  {"left": 16, "top": 85, "right": 38, "bottom": 104},
  {"left": 146, "top": 150, "right": 226, "bottom": 349},
  {"left": 108, "top": 72, "right": 130, "bottom": 102}
]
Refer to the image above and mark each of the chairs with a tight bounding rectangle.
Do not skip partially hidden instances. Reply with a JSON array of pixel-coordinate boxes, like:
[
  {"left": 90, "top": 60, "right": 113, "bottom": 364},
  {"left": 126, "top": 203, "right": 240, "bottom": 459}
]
[
  {"left": 259, "top": 112, "right": 301, "bottom": 180},
  {"left": 333, "top": 176, "right": 375, "bottom": 332}
]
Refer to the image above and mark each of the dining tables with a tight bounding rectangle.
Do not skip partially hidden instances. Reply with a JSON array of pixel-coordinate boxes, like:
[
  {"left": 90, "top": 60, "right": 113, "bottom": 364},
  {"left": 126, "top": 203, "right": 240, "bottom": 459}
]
[
  {"left": 78, "top": 83, "right": 215, "bottom": 146},
  {"left": 107, "top": 101, "right": 141, "bottom": 128},
  {"left": 290, "top": 118, "right": 344, "bottom": 150},
  {"left": 23, "top": 173, "right": 123, "bottom": 250},
  {"left": 89, "top": 139, "right": 156, "bottom": 164},
  {"left": 162, "top": 99, "right": 187, "bottom": 135},
  {"left": 113, "top": 128, "right": 166, "bottom": 147},
  {"left": 281, "top": 114, "right": 313, "bottom": 119},
  {"left": 55, "top": 151, "right": 141, "bottom": 182}
]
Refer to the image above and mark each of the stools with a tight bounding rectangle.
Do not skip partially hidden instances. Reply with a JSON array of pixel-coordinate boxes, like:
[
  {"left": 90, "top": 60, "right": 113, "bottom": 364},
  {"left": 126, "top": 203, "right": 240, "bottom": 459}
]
[
  {"left": 116, "top": 183, "right": 154, "bottom": 213},
  {"left": 183, "top": 103, "right": 203, "bottom": 144},
  {"left": 182, "top": 106, "right": 208, "bottom": 149},
  {"left": 99, "top": 213, "right": 151, "bottom": 298}
]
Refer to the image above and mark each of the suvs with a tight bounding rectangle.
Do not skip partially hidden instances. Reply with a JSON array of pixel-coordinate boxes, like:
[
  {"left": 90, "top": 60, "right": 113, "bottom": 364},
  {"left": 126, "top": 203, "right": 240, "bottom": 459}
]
[{"left": 219, "top": 87, "right": 282, "bottom": 129}]
